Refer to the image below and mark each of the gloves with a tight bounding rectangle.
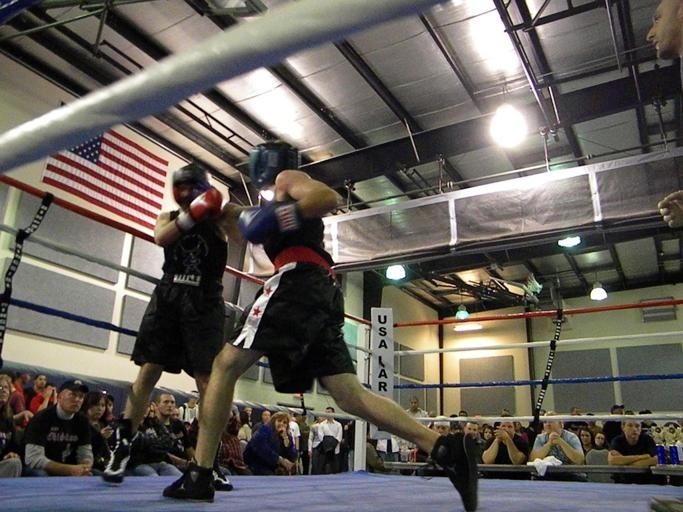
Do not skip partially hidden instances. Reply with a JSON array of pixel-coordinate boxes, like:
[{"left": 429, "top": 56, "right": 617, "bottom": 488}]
[
  {"left": 237, "top": 205, "right": 298, "bottom": 244},
  {"left": 178, "top": 187, "right": 222, "bottom": 228}
]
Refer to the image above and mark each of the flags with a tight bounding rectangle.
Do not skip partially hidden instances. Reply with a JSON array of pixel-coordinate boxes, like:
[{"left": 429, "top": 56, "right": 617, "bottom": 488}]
[{"left": 40, "top": 103, "right": 169, "bottom": 237}]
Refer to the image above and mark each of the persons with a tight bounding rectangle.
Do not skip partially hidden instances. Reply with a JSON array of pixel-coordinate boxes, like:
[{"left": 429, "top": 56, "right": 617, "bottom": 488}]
[
  {"left": 1, "top": 371, "right": 200, "bottom": 486},
  {"left": 647, "top": 2, "right": 683, "bottom": 512},
  {"left": 366, "top": 395, "right": 683, "bottom": 488},
  {"left": 218, "top": 405, "right": 355, "bottom": 477},
  {"left": 164, "top": 141, "right": 482, "bottom": 512},
  {"left": 101, "top": 168, "right": 233, "bottom": 493}
]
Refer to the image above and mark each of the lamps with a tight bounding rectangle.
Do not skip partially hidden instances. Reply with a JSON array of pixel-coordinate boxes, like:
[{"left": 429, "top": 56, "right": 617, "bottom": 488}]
[
  {"left": 589, "top": 265, "right": 606, "bottom": 301},
  {"left": 487, "top": 86, "right": 529, "bottom": 148},
  {"left": 451, "top": 289, "right": 479, "bottom": 331}
]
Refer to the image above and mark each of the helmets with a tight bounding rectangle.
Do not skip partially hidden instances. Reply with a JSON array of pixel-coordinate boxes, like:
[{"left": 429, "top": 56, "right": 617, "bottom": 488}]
[
  {"left": 249, "top": 140, "right": 302, "bottom": 188},
  {"left": 173, "top": 164, "right": 209, "bottom": 206}
]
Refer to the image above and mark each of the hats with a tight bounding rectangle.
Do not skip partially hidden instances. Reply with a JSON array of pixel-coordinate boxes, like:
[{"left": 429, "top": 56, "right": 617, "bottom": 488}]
[
  {"left": 60, "top": 380, "right": 88, "bottom": 393},
  {"left": 434, "top": 416, "right": 451, "bottom": 426}
]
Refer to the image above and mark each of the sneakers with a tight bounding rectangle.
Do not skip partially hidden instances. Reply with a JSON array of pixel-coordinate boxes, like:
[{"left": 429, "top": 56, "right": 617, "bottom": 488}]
[
  {"left": 163, "top": 463, "right": 232, "bottom": 499},
  {"left": 442, "top": 433, "right": 477, "bottom": 512},
  {"left": 103, "top": 419, "right": 139, "bottom": 482}
]
